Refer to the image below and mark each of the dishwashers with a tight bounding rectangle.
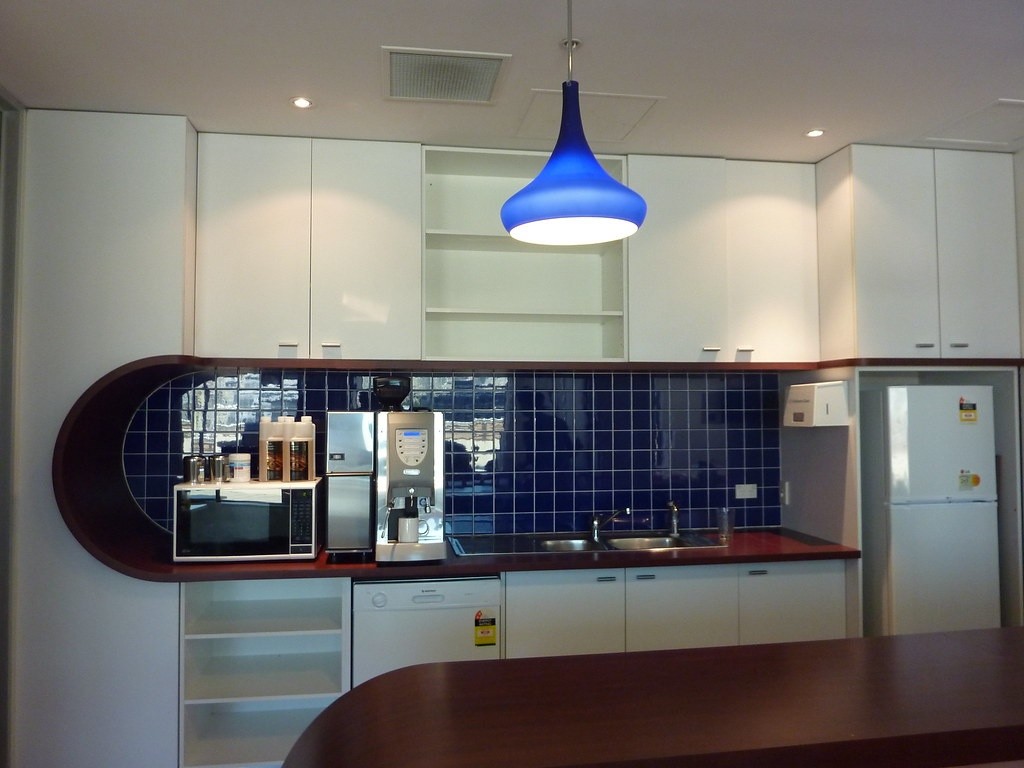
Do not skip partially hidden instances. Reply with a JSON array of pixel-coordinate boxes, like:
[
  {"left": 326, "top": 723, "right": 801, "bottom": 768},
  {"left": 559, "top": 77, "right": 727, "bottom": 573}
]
[{"left": 351, "top": 575, "right": 503, "bottom": 693}]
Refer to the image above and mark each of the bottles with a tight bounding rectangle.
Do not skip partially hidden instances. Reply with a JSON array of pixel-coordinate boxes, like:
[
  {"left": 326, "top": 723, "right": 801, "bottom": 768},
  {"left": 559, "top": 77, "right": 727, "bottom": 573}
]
[{"left": 229, "top": 453, "right": 251, "bottom": 482}]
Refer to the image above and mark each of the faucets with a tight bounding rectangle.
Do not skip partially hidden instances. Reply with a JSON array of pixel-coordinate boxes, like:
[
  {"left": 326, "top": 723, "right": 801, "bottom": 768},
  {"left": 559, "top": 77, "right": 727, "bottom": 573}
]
[{"left": 592, "top": 507, "right": 631, "bottom": 543}]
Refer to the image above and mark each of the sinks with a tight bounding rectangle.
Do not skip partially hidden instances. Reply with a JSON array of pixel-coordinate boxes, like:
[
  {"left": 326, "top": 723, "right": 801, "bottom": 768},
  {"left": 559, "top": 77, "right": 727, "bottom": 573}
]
[
  {"left": 607, "top": 536, "right": 693, "bottom": 549},
  {"left": 543, "top": 538, "right": 594, "bottom": 552}
]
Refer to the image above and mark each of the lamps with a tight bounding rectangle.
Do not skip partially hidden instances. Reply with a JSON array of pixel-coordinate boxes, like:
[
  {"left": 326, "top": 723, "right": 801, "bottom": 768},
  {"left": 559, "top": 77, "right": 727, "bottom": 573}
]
[{"left": 502, "top": 2, "right": 649, "bottom": 245}]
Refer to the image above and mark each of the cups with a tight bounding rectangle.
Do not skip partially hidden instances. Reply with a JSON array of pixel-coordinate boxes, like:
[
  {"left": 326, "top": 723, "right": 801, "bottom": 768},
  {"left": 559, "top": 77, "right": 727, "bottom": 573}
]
[
  {"left": 716, "top": 508, "right": 735, "bottom": 543},
  {"left": 397, "top": 518, "right": 429, "bottom": 543},
  {"left": 182, "top": 454, "right": 206, "bottom": 482},
  {"left": 207, "top": 454, "right": 229, "bottom": 482}
]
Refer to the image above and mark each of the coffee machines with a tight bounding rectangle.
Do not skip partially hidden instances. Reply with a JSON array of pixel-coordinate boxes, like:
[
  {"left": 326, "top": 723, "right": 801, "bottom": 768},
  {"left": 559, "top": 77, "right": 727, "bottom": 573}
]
[{"left": 374, "top": 410, "right": 446, "bottom": 564}]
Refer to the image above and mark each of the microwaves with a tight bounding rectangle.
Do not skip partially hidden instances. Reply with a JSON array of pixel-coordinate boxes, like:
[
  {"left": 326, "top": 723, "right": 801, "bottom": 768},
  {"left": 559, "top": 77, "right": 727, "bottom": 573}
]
[{"left": 172, "top": 477, "right": 324, "bottom": 562}]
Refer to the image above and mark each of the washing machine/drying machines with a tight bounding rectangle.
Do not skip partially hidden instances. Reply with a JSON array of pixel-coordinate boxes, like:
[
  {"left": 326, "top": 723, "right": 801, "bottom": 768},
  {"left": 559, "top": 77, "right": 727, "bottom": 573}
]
[{"left": 350, "top": 581, "right": 500, "bottom": 685}]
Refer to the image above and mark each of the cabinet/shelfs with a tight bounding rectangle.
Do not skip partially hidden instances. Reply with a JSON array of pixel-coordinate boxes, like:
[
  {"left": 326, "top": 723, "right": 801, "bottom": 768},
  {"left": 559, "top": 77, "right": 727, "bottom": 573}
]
[
  {"left": 854, "top": 146, "right": 1020, "bottom": 361},
  {"left": 179, "top": 577, "right": 351, "bottom": 767},
  {"left": 420, "top": 146, "right": 629, "bottom": 362},
  {"left": 186, "top": 137, "right": 416, "bottom": 361},
  {"left": 740, "top": 561, "right": 848, "bottom": 646},
  {"left": 629, "top": 158, "right": 827, "bottom": 366},
  {"left": 502, "top": 564, "right": 739, "bottom": 658}
]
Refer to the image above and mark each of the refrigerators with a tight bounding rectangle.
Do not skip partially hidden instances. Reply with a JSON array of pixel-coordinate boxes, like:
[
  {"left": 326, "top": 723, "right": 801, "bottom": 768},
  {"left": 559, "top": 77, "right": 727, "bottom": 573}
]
[{"left": 860, "top": 386, "right": 1003, "bottom": 638}]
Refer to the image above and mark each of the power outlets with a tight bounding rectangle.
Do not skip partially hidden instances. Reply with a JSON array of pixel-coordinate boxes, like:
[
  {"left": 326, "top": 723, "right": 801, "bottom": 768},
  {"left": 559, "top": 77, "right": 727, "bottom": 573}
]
[
  {"left": 736, "top": 484, "right": 757, "bottom": 499},
  {"left": 780, "top": 481, "right": 790, "bottom": 505}
]
